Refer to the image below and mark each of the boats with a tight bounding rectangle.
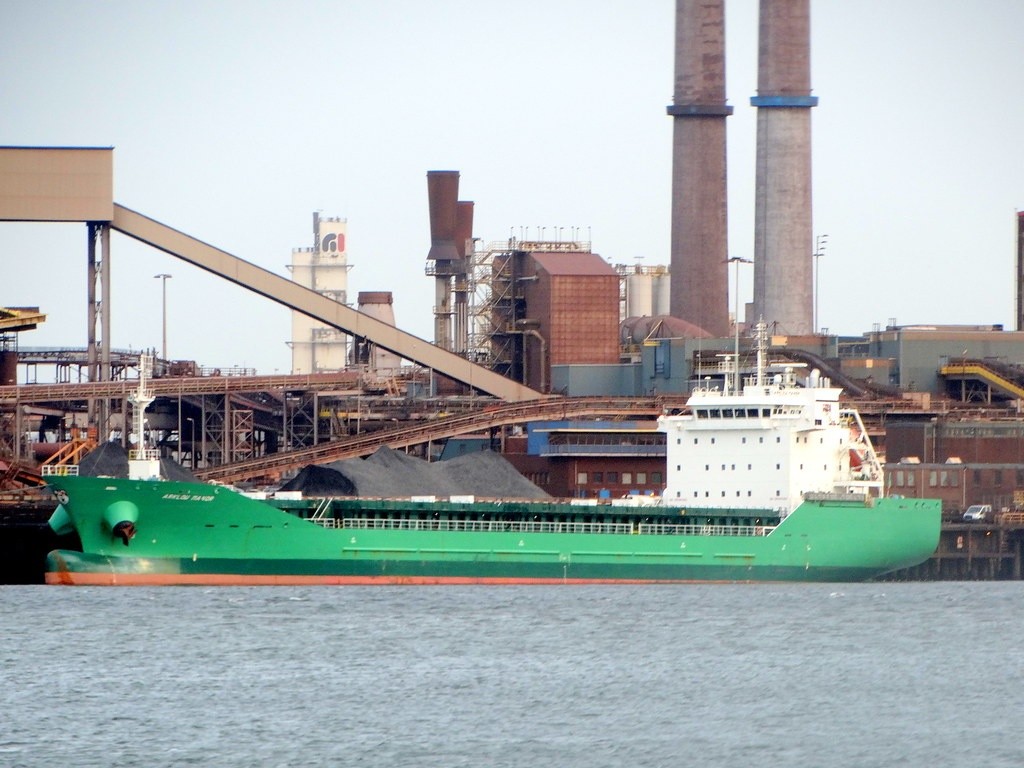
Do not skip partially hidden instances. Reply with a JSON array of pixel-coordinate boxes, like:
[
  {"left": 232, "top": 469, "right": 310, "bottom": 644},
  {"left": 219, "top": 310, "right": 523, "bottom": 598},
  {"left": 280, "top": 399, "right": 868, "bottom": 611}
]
[{"left": 41, "top": 313, "right": 944, "bottom": 585}]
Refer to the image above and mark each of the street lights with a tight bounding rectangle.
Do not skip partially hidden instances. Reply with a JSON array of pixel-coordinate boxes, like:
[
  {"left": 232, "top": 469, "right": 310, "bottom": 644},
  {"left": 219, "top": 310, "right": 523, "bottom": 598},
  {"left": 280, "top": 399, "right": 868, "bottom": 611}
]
[
  {"left": 155, "top": 274, "right": 172, "bottom": 374},
  {"left": 731, "top": 257, "right": 750, "bottom": 392}
]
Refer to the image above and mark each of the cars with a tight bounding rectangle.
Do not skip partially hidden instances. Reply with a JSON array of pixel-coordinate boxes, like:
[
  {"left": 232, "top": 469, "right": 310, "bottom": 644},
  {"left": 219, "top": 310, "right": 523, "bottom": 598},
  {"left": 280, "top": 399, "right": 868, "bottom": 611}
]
[{"left": 963, "top": 505, "right": 993, "bottom": 521}]
